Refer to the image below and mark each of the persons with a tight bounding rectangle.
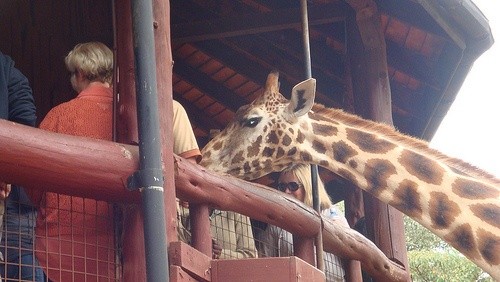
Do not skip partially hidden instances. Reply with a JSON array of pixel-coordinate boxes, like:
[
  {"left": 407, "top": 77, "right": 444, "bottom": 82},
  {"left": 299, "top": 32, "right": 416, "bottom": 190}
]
[
  {"left": 173, "top": 98, "right": 202, "bottom": 165},
  {"left": 259, "top": 163, "right": 363, "bottom": 282},
  {"left": 16, "top": 41, "right": 122, "bottom": 282},
  {"left": 0, "top": 49, "right": 45, "bottom": 282},
  {"left": 175, "top": 199, "right": 258, "bottom": 259}
]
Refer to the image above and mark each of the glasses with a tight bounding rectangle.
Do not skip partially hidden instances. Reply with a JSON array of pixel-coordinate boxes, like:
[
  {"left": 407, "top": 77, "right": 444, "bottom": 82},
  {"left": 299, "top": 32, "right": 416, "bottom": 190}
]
[{"left": 278, "top": 182, "right": 302, "bottom": 192}]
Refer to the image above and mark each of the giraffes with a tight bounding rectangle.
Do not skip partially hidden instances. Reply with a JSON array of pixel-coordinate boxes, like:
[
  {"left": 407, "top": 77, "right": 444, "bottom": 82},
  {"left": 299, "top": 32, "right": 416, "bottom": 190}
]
[{"left": 198, "top": 68, "right": 500, "bottom": 282}]
